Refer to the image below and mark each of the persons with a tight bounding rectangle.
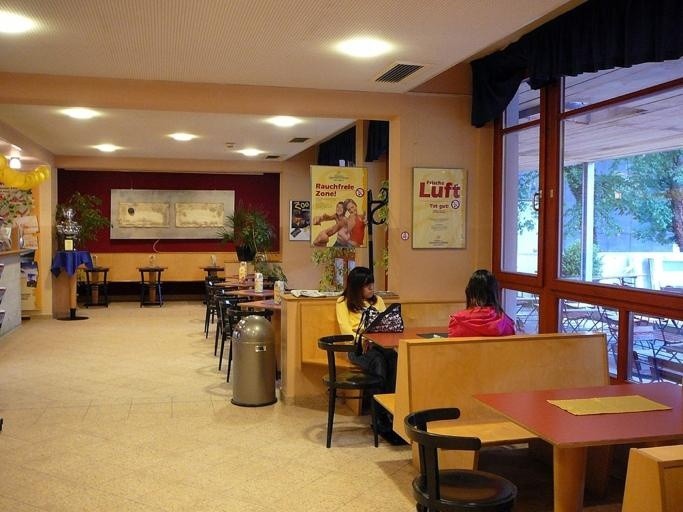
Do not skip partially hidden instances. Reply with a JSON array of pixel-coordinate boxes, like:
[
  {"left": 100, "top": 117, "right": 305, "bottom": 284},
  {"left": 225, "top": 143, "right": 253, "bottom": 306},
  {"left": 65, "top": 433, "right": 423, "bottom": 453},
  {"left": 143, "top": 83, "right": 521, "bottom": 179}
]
[
  {"left": 335, "top": 265, "right": 399, "bottom": 434},
  {"left": 446, "top": 269, "right": 515, "bottom": 337},
  {"left": 315, "top": 202, "right": 349, "bottom": 246},
  {"left": 312, "top": 196, "right": 366, "bottom": 246}
]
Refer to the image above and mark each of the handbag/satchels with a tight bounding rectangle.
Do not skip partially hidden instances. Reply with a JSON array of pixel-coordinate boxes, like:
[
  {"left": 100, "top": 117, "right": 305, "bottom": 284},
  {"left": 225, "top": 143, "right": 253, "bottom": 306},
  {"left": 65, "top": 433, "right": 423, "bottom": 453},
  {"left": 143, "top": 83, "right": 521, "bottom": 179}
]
[{"left": 354, "top": 303, "right": 404, "bottom": 355}]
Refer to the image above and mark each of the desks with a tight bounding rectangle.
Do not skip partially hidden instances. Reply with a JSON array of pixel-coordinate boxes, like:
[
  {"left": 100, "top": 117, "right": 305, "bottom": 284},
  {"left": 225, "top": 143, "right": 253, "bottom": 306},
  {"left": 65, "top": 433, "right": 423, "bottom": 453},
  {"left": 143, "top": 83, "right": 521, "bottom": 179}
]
[{"left": 50, "top": 250, "right": 93, "bottom": 320}]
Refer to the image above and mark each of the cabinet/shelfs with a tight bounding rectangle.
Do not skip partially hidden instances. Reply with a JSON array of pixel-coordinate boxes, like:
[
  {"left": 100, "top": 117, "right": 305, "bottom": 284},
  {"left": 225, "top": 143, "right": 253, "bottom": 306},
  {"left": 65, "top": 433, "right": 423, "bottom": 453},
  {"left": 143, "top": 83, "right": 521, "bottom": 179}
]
[{"left": 0, "top": 248, "right": 23, "bottom": 338}]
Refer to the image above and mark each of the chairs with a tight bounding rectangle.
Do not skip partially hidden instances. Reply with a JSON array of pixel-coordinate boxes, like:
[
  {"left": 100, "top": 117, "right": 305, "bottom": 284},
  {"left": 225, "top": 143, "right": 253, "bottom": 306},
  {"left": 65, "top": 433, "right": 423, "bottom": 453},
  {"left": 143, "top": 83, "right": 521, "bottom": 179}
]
[
  {"left": 203, "top": 275, "right": 277, "bottom": 384},
  {"left": 404, "top": 409, "right": 518, "bottom": 512},
  {"left": 317, "top": 335, "right": 383, "bottom": 449}
]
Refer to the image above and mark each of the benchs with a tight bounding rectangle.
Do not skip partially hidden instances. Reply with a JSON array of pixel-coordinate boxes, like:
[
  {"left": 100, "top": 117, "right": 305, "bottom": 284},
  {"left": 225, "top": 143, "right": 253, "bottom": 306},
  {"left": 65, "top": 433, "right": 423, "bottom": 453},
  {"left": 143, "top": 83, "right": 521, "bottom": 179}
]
[
  {"left": 374, "top": 330, "right": 615, "bottom": 498},
  {"left": 579, "top": 444, "right": 683, "bottom": 512}
]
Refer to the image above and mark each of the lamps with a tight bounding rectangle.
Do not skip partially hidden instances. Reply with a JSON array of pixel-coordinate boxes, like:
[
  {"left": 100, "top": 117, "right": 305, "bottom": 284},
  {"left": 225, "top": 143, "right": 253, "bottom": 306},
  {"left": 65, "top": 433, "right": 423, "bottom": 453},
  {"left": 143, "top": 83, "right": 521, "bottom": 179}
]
[{"left": 9, "top": 156, "right": 21, "bottom": 169}]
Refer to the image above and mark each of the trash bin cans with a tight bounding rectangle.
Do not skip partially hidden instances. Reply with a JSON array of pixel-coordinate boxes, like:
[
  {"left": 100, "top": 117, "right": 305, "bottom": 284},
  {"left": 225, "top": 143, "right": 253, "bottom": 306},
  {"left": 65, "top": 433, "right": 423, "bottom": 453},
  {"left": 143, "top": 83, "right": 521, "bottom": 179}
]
[{"left": 230, "top": 315, "right": 277, "bottom": 407}]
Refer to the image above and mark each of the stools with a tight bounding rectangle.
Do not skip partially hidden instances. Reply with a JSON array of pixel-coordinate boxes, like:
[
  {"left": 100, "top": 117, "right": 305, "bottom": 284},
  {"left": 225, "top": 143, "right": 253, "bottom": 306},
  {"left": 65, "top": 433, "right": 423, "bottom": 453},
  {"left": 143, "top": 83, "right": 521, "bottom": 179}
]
[
  {"left": 84, "top": 267, "right": 110, "bottom": 307},
  {"left": 136, "top": 265, "right": 168, "bottom": 308}
]
